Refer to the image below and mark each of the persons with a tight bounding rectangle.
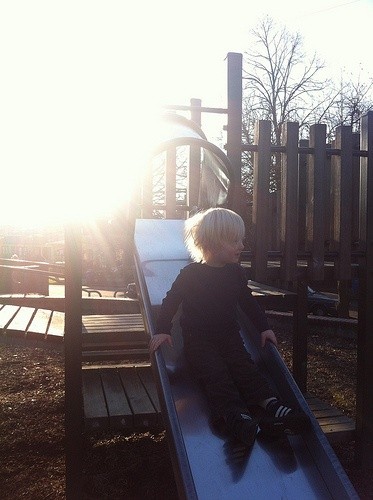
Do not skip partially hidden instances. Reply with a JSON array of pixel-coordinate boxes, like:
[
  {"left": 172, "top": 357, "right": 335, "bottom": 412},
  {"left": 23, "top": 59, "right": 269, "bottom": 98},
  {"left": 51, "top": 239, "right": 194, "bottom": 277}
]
[{"left": 149, "top": 207, "right": 301, "bottom": 441}]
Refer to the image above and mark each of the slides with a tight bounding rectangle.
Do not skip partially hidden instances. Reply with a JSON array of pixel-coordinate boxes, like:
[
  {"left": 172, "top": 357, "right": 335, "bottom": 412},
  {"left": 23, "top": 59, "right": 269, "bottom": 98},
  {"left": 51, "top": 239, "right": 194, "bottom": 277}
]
[{"left": 132, "top": 218, "right": 361, "bottom": 500}]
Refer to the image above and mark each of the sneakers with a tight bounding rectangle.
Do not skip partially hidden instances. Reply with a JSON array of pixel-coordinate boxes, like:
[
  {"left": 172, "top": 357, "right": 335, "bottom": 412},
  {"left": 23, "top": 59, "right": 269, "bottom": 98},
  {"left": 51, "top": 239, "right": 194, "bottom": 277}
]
[
  {"left": 230, "top": 409, "right": 261, "bottom": 453},
  {"left": 265, "top": 400, "right": 306, "bottom": 438}
]
